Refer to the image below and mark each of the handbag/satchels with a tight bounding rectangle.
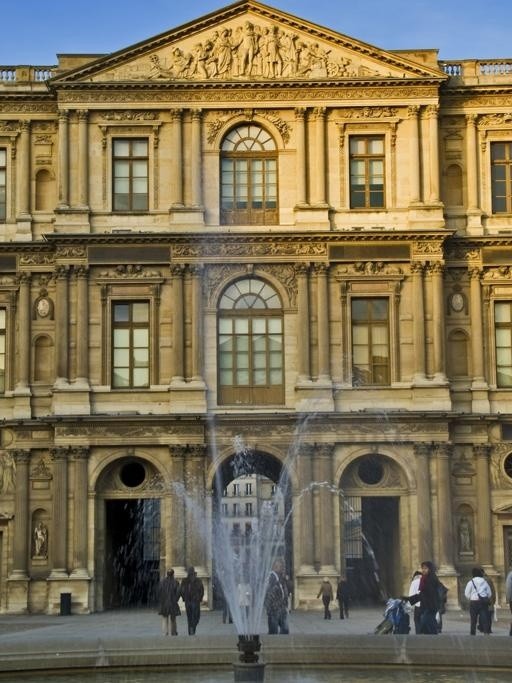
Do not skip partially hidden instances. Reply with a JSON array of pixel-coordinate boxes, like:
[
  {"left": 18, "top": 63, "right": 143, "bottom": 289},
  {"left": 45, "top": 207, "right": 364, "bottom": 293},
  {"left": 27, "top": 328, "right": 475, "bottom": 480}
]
[
  {"left": 479, "top": 596, "right": 491, "bottom": 605},
  {"left": 436, "top": 581, "right": 448, "bottom": 602}
]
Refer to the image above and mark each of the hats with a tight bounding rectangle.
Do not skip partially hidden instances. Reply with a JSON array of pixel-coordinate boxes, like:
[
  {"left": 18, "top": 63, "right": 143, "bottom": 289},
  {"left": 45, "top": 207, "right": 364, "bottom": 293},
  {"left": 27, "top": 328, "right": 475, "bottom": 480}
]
[{"left": 323, "top": 576, "right": 329, "bottom": 582}]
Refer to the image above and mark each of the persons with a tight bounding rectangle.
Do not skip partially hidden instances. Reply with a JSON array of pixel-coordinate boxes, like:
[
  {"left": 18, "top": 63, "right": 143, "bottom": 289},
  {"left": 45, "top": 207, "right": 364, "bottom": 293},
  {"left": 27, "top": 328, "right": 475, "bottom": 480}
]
[
  {"left": 150, "top": 21, "right": 330, "bottom": 79},
  {"left": 464, "top": 568, "right": 492, "bottom": 634},
  {"left": 399, "top": 561, "right": 448, "bottom": 634},
  {"left": 504, "top": 572, "right": 512, "bottom": 635},
  {"left": 477, "top": 569, "right": 496, "bottom": 632},
  {"left": 459, "top": 516, "right": 472, "bottom": 551},
  {"left": 337, "top": 576, "right": 350, "bottom": 619},
  {"left": 222, "top": 560, "right": 293, "bottom": 634},
  {"left": 317, "top": 576, "right": 333, "bottom": 620},
  {"left": 34, "top": 522, "right": 46, "bottom": 556},
  {"left": 157, "top": 569, "right": 181, "bottom": 635},
  {"left": 181, "top": 567, "right": 204, "bottom": 634}
]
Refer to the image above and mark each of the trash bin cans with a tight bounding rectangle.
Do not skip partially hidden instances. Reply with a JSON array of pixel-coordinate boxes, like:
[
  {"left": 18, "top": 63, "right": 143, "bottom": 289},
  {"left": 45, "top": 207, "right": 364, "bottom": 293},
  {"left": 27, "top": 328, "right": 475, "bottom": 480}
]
[{"left": 60, "top": 593, "right": 71, "bottom": 615}]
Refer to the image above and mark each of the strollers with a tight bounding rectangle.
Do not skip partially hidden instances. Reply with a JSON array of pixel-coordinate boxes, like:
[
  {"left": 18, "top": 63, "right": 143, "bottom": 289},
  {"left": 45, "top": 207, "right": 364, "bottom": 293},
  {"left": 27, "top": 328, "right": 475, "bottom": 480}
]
[{"left": 375, "top": 598, "right": 411, "bottom": 633}]
